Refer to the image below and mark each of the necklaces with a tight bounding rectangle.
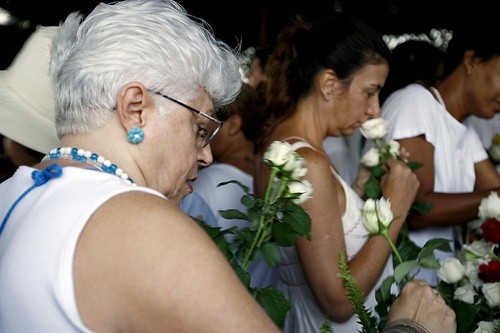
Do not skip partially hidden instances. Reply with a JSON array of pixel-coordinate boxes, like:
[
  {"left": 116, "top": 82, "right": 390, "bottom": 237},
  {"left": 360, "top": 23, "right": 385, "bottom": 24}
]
[{"left": 41, "top": 147, "right": 136, "bottom": 184}]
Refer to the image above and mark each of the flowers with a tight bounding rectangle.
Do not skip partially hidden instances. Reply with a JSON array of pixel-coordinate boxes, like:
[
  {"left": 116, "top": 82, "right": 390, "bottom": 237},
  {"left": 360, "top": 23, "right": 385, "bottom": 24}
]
[
  {"left": 196, "top": 139, "right": 312, "bottom": 331},
  {"left": 320, "top": 119, "right": 500, "bottom": 333}
]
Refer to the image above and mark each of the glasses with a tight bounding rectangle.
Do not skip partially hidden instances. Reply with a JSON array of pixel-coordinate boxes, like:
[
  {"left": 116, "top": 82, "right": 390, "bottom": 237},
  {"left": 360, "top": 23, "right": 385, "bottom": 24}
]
[{"left": 112, "top": 89, "right": 223, "bottom": 148}]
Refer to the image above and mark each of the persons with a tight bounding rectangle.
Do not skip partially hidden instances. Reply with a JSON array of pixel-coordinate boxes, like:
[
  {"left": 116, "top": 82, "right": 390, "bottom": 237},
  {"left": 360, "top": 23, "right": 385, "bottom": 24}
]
[
  {"left": 252, "top": 15, "right": 420, "bottom": 333},
  {"left": 359, "top": 27, "right": 500, "bottom": 290},
  {"left": 0, "top": 0, "right": 500, "bottom": 333},
  {"left": 0, "top": 27, "right": 62, "bottom": 184},
  {"left": 0, "top": 1, "right": 459, "bottom": 333}
]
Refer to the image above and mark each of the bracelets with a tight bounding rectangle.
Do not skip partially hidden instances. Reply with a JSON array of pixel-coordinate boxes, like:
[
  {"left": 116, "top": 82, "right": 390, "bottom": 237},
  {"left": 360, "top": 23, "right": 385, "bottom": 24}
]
[{"left": 384, "top": 320, "right": 431, "bottom": 333}]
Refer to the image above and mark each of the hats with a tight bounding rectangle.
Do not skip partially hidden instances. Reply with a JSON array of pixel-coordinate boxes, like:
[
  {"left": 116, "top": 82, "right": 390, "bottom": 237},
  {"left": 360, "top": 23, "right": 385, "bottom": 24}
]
[{"left": 0, "top": 25, "right": 61, "bottom": 154}]
